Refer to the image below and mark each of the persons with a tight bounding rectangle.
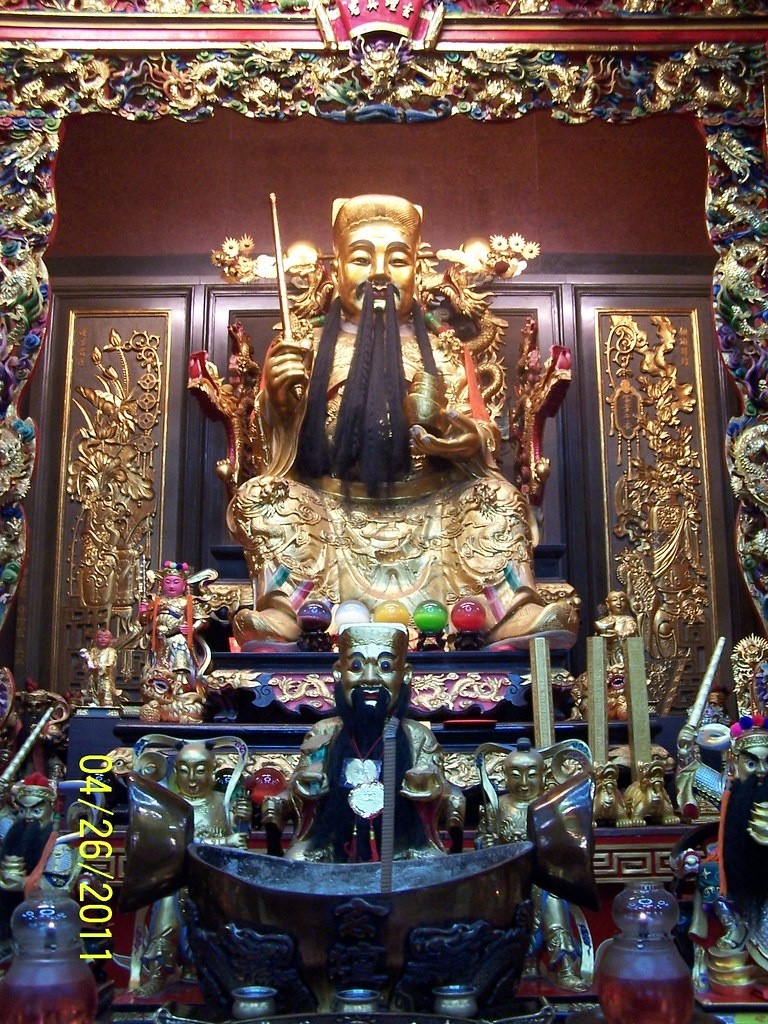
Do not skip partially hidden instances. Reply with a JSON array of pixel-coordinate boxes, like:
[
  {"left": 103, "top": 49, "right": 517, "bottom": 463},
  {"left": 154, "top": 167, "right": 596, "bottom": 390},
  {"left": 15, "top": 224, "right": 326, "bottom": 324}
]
[
  {"left": 232, "top": 192, "right": 578, "bottom": 651},
  {"left": 0, "top": 773, "right": 77, "bottom": 968},
  {"left": 697, "top": 728, "right": 768, "bottom": 952},
  {"left": 138, "top": 560, "right": 209, "bottom": 685},
  {"left": 136, "top": 741, "right": 253, "bottom": 998},
  {"left": 81, "top": 628, "right": 118, "bottom": 702},
  {"left": 596, "top": 590, "right": 638, "bottom": 666},
  {"left": 288, "top": 625, "right": 445, "bottom": 862},
  {"left": 473, "top": 741, "right": 589, "bottom": 992}
]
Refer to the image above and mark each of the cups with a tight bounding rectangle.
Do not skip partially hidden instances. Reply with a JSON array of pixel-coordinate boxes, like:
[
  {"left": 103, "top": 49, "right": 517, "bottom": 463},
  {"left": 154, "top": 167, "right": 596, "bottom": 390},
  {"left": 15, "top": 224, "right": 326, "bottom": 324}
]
[
  {"left": 432, "top": 984, "right": 477, "bottom": 1019},
  {"left": 334, "top": 989, "right": 382, "bottom": 1015},
  {"left": 231, "top": 987, "right": 278, "bottom": 1019}
]
